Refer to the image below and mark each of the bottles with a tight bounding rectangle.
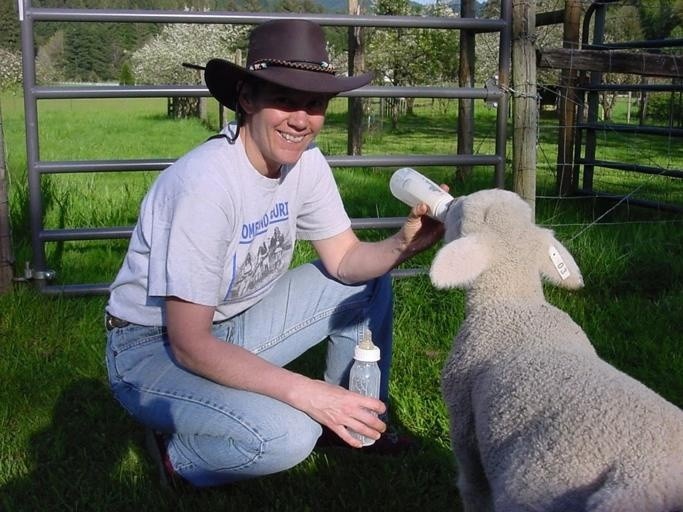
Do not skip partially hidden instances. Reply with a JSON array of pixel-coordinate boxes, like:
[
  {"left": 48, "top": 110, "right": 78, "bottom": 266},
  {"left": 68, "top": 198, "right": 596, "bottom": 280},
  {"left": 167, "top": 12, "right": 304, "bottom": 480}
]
[
  {"left": 343, "top": 329, "right": 384, "bottom": 449},
  {"left": 388, "top": 167, "right": 453, "bottom": 223}
]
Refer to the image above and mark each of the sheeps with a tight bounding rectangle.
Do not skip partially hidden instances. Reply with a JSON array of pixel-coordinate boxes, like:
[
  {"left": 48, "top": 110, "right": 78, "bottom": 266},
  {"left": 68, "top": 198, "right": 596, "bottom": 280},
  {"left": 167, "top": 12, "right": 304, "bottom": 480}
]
[{"left": 428, "top": 187, "right": 683, "bottom": 512}]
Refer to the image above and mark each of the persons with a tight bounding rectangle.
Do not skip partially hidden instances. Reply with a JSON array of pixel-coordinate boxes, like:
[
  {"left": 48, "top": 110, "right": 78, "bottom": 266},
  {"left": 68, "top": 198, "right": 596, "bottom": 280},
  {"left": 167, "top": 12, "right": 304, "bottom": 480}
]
[{"left": 105, "top": 76, "right": 450, "bottom": 491}]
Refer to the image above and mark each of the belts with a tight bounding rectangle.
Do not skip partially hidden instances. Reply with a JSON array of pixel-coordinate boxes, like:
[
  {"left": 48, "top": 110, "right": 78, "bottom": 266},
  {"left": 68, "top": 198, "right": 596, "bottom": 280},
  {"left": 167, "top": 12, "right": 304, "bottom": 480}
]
[{"left": 104, "top": 314, "right": 130, "bottom": 331}]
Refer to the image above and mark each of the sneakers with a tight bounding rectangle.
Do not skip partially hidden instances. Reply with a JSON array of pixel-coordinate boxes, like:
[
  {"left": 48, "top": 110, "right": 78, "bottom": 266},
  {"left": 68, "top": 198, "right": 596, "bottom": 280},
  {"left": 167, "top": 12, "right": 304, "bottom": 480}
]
[
  {"left": 144, "top": 426, "right": 182, "bottom": 494},
  {"left": 362, "top": 433, "right": 411, "bottom": 451}
]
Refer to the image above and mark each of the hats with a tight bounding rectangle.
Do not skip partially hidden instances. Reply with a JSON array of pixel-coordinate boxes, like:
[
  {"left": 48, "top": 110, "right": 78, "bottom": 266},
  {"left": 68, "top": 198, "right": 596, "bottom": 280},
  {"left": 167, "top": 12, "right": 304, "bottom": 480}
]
[{"left": 203, "top": 20, "right": 376, "bottom": 113}]
